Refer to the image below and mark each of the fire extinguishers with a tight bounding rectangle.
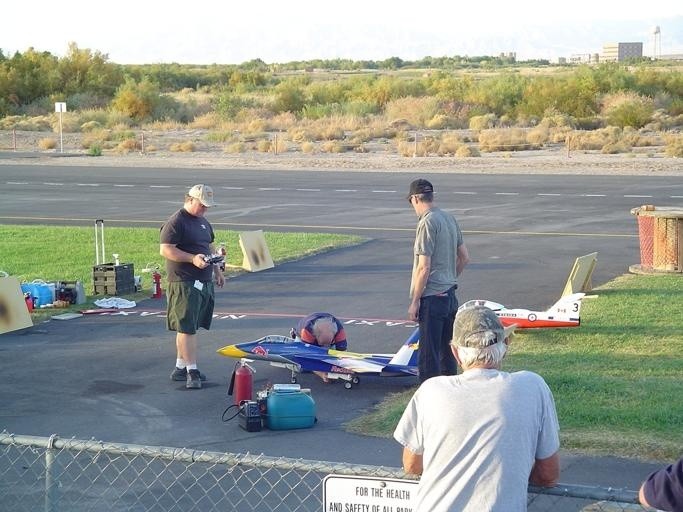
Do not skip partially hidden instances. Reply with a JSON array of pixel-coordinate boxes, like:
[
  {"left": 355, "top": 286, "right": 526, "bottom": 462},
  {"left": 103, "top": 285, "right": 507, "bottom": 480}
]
[
  {"left": 152, "top": 267, "right": 162, "bottom": 298},
  {"left": 227, "top": 357, "right": 256, "bottom": 409},
  {"left": 23, "top": 291, "right": 33, "bottom": 313}
]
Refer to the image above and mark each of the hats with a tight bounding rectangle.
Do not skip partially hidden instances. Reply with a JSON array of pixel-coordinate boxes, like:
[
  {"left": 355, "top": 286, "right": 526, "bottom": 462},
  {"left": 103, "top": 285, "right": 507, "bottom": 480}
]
[
  {"left": 452, "top": 305, "right": 504, "bottom": 348},
  {"left": 188, "top": 184, "right": 219, "bottom": 209},
  {"left": 407, "top": 177, "right": 434, "bottom": 197}
]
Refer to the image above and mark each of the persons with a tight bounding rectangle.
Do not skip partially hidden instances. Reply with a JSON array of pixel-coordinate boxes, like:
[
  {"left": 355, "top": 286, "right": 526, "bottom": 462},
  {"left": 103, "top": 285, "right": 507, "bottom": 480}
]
[
  {"left": 406, "top": 179, "right": 469, "bottom": 385},
  {"left": 160, "top": 184, "right": 224, "bottom": 388},
  {"left": 290, "top": 313, "right": 347, "bottom": 382},
  {"left": 639, "top": 456, "right": 683, "bottom": 512},
  {"left": 393, "top": 305, "right": 561, "bottom": 512}
]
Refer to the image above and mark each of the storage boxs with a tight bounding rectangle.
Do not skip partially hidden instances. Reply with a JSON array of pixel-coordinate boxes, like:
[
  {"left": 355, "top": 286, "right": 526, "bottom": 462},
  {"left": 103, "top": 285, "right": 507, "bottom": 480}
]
[{"left": 92, "top": 261, "right": 135, "bottom": 295}]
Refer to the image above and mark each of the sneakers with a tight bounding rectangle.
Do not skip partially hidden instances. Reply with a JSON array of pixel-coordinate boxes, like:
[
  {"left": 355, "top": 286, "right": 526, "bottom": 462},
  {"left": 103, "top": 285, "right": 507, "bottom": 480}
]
[{"left": 171, "top": 365, "right": 208, "bottom": 388}]
